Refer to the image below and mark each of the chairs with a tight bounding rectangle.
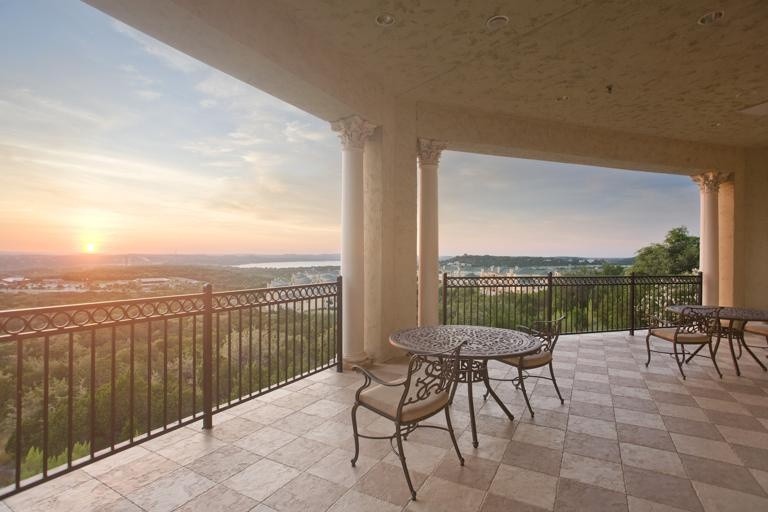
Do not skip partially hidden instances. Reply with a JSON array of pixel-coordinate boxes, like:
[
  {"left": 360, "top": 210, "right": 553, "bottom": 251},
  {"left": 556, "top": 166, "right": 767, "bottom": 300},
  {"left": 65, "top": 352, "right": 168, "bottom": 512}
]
[
  {"left": 736, "top": 319, "right": 768, "bottom": 360},
  {"left": 645, "top": 306, "right": 725, "bottom": 379},
  {"left": 350, "top": 340, "right": 467, "bottom": 501},
  {"left": 483, "top": 316, "right": 567, "bottom": 417}
]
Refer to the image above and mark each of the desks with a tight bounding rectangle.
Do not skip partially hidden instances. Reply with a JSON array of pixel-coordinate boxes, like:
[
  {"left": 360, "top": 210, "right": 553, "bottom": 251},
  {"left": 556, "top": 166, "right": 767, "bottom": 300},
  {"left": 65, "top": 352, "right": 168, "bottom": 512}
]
[
  {"left": 389, "top": 324, "right": 541, "bottom": 449},
  {"left": 664, "top": 307, "right": 768, "bottom": 376}
]
